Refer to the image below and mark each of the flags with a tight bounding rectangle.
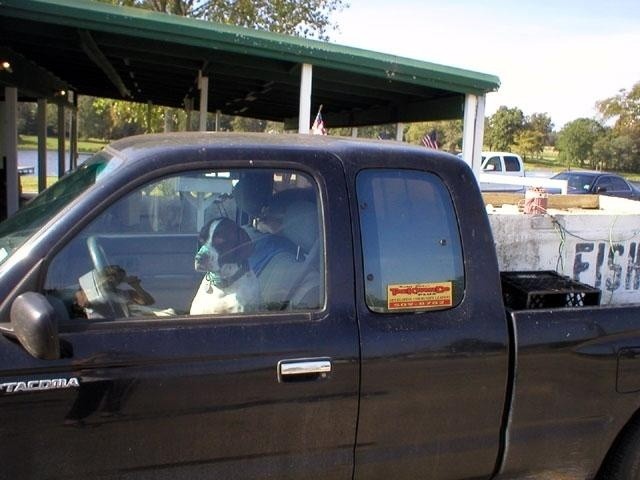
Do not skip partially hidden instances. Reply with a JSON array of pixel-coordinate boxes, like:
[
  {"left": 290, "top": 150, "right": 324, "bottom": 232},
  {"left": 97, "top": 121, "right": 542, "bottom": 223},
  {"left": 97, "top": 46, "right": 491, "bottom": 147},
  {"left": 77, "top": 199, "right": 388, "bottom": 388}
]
[
  {"left": 418, "top": 130, "right": 438, "bottom": 151},
  {"left": 376, "top": 133, "right": 384, "bottom": 140},
  {"left": 312, "top": 112, "right": 327, "bottom": 135}
]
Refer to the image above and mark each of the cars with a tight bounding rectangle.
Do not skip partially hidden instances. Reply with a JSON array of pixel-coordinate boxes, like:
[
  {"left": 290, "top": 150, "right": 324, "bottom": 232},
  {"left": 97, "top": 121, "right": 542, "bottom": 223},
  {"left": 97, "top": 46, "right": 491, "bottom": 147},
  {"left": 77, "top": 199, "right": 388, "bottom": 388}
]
[{"left": 515, "top": 170, "right": 639, "bottom": 202}]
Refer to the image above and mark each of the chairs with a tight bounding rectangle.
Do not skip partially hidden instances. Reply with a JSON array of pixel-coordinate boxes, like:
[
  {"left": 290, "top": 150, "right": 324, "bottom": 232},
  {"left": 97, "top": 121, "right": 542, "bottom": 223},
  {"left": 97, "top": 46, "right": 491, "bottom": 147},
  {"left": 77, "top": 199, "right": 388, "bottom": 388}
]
[
  {"left": 232, "top": 174, "right": 278, "bottom": 274},
  {"left": 255, "top": 200, "right": 320, "bottom": 311}
]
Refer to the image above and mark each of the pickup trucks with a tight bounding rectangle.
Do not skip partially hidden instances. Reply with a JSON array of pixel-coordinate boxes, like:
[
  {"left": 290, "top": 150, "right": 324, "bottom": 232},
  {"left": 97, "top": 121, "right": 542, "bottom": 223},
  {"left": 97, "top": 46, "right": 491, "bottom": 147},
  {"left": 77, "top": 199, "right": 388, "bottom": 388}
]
[
  {"left": 456, "top": 150, "right": 560, "bottom": 193},
  {"left": 0, "top": 131, "right": 640, "bottom": 480}
]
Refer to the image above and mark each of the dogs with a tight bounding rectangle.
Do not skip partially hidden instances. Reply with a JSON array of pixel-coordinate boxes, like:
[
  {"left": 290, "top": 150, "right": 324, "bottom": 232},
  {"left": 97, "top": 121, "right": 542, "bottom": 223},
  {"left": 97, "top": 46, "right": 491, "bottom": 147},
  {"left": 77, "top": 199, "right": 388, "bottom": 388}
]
[{"left": 188, "top": 217, "right": 260, "bottom": 316}]
